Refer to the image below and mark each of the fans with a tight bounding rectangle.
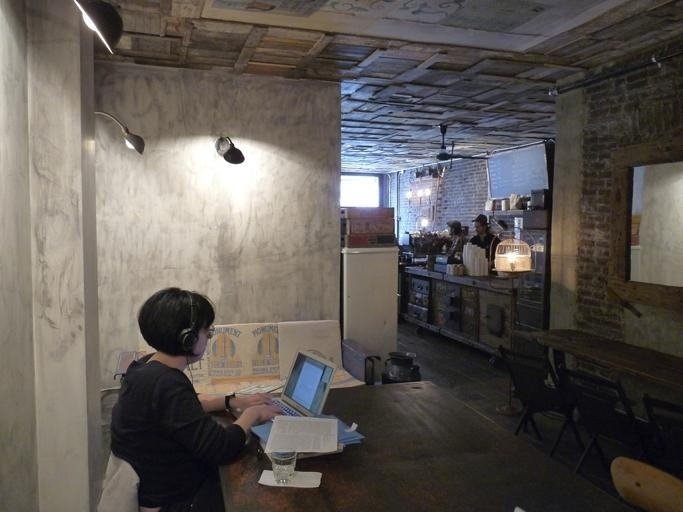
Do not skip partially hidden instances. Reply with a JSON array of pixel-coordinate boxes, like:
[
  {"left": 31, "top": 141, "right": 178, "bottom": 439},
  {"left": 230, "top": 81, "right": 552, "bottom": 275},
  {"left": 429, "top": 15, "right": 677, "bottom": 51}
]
[{"left": 402, "top": 123, "right": 491, "bottom": 162}]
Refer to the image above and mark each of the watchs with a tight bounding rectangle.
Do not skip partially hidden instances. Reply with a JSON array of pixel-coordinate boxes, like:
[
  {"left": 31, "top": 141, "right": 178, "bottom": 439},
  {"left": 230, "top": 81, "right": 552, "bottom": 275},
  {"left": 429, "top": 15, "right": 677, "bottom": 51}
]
[{"left": 224, "top": 392, "right": 235, "bottom": 412}]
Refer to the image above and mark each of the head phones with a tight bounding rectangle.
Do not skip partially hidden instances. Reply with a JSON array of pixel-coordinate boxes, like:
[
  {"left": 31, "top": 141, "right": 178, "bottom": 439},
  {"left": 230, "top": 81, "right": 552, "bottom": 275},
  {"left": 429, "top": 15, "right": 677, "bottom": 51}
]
[{"left": 178, "top": 290, "right": 199, "bottom": 351}]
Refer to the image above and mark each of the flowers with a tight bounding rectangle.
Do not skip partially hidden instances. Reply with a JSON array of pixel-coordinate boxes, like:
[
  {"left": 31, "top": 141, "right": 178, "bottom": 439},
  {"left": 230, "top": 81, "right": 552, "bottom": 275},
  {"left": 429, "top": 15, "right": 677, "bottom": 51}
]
[{"left": 409, "top": 232, "right": 454, "bottom": 254}]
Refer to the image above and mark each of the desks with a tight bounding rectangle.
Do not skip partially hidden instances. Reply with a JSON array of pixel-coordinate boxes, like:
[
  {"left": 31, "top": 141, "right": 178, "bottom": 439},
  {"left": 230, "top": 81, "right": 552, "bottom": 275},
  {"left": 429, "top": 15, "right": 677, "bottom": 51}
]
[
  {"left": 505, "top": 329, "right": 683, "bottom": 395},
  {"left": 208, "top": 379, "right": 639, "bottom": 512}
]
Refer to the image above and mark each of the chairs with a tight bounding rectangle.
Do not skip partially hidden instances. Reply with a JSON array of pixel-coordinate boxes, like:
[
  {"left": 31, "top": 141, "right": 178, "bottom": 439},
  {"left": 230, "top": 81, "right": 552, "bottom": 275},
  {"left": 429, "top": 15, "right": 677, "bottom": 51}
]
[
  {"left": 641, "top": 392, "right": 683, "bottom": 480},
  {"left": 497, "top": 343, "right": 585, "bottom": 458},
  {"left": 553, "top": 363, "right": 656, "bottom": 500}
]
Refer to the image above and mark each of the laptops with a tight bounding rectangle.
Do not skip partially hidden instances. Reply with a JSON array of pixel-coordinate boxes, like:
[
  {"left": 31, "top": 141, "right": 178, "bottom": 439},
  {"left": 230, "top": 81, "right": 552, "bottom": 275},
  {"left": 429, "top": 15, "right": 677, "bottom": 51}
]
[{"left": 231, "top": 349, "right": 337, "bottom": 426}]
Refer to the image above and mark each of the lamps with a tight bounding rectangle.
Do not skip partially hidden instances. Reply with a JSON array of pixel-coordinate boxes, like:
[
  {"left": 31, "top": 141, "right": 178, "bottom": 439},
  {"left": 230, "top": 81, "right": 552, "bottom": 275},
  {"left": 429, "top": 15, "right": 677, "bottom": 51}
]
[
  {"left": 73, "top": 0, "right": 124, "bottom": 55},
  {"left": 94, "top": 111, "right": 145, "bottom": 156},
  {"left": 214, "top": 136, "right": 245, "bottom": 164}
]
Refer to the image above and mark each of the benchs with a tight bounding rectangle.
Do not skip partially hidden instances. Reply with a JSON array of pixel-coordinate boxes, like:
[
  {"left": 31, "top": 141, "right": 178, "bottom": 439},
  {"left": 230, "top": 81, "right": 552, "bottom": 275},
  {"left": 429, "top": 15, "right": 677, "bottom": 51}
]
[{"left": 100, "top": 319, "right": 383, "bottom": 403}]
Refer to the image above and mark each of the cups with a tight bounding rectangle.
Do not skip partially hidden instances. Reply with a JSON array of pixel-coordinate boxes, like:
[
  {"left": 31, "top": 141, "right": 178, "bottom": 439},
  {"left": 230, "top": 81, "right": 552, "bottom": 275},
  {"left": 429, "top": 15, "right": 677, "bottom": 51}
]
[
  {"left": 382, "top": 350, "right": 416, "bottom": 383},
  {"left": 461, "top": 243, "right": 489, "bottom": 277},
  {"left": 271, "top": 446, "right": 297, "bottom": 484}
]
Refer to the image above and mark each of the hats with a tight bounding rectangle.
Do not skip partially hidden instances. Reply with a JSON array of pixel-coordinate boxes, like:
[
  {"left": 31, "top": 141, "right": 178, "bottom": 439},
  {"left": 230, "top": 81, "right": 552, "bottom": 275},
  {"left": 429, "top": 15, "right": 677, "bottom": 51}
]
[
  {"left": 471, "top": 213, "right": 491, "bottom": 226},
  {"left": 446, "top": 220, "right": 461, "bottom": 228}
]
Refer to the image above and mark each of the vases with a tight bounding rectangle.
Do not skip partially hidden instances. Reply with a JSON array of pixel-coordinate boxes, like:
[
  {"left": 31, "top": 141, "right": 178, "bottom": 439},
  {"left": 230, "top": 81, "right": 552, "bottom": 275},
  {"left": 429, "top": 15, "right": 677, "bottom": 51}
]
[{"left": 427, "top": 253, "right": 436, "bottom": 271}]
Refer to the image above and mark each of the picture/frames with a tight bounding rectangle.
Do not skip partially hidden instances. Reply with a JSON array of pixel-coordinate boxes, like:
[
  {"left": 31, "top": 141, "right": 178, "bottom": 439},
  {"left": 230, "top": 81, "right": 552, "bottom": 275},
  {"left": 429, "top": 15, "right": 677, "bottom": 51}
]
[{"left": 485, "top": 139, "right": 550, "bottom": 200}]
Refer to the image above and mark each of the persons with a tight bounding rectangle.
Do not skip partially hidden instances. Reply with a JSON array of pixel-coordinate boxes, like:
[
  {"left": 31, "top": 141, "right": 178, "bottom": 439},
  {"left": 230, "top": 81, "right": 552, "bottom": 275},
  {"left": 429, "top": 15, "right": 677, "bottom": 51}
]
[
  {"left": 468, "top": 214, "right": 501, "bottom": 261},
  {"left": 110, "top": 287, "right": 283, "bottom": 512},
  {"left": 445, "top": 220, "right": 468, "bottom": 257}
]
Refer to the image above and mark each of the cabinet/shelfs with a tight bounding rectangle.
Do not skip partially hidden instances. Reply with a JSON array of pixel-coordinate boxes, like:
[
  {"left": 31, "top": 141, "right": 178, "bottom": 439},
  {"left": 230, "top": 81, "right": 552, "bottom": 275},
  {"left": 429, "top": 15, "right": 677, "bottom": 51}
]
[{"left": 341, "top": 247, "right": 400, "bottom": 382}]
